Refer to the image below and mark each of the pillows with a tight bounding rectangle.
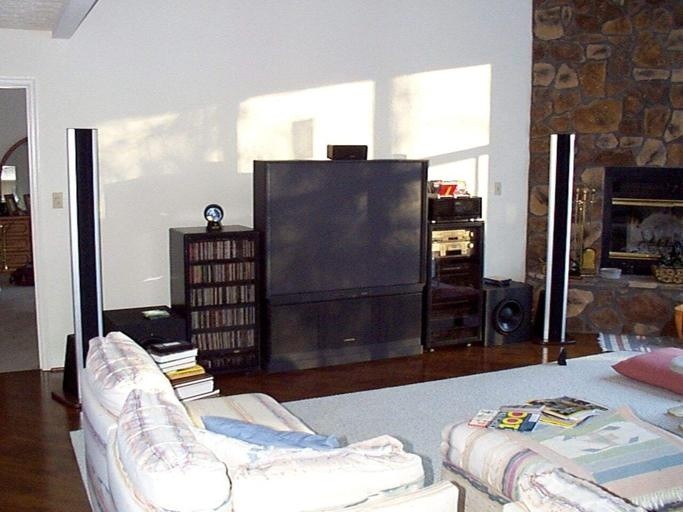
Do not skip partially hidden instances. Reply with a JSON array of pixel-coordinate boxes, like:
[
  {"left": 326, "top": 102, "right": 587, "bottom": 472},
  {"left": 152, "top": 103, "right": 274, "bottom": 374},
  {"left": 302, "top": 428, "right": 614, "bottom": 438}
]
[
  {"left": 612, "top": 344, "right": 683, "bottom": 396},
  {"left": 200, "top": 412, "right": 341, "bottom": 456}
]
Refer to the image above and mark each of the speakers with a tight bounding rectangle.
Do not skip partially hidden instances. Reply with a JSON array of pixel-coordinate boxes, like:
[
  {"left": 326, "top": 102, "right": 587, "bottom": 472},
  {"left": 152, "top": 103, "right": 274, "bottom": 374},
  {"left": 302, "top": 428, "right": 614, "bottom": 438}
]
[
  {"left": 483, "top": 280, "right": 533, "bottom": 348},
  {"left": 66, "top": 127, "right": 104, "bottom": 404},
  {"left": 532, "top": 131, "right": 576, "bottom": 346},
  {"left": 102, "top": 304, "right": 189, "bottom": 353}
]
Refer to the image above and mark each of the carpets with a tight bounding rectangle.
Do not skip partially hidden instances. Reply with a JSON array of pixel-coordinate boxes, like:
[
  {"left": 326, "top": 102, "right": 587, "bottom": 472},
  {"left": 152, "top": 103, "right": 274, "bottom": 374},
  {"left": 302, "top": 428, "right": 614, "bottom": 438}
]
[
  {"left": 69, "top": 350, "right": 683, "bottom": 512},
  {"left": 595, "top": 330, "right": 683, "bottom": 355}
]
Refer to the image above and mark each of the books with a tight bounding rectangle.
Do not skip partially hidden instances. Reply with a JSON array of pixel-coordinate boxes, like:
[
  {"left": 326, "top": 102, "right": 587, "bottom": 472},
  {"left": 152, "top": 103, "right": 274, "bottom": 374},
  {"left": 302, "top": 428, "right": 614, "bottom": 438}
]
[
  {"left": 466, "top": 394, "right": 608, "bottom": 434},
  {"left": 147, "top": 339, "right": 221, "bottom": 403},
  {"left": 189, "top": 239, "right": 258, "bottom": 370},
  {"left": 426, "top": 178, "right": 458, "bottom": 198}
]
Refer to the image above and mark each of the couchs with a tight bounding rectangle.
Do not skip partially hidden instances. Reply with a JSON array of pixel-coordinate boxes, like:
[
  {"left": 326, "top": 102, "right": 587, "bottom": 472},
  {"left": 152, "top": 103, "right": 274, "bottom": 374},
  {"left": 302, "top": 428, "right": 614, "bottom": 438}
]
[
  {"left": 442, "top": 391, "right": 683, "bottom": 512},
  {"left": 77, "top": 328, "right": 424, "bottom": 512}
]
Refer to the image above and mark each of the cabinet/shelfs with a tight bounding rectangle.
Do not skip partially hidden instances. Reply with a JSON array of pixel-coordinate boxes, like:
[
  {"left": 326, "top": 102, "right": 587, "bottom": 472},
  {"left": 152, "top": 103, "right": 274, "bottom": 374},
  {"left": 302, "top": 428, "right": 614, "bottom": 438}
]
[
  {"left": 169, "top": 225, "right": 266, "bottom": 375},
  {"left": 426, "top": 221, "right": 487, "bottom": 349},
  {"left": 0, "top": 216, "right": 32, "bottom": 274}
]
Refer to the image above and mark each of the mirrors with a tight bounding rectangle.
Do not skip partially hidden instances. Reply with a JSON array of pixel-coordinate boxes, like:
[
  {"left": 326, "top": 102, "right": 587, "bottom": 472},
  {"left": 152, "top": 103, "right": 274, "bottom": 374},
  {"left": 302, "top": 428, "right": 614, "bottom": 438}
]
[{"left": 0, "top": 137, "right": 30, "bottom": 216}]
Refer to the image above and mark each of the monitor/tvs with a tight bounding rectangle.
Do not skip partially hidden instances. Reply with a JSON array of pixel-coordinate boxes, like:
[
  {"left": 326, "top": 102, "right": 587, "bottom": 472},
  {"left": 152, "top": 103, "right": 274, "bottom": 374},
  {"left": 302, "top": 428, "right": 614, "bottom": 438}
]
[{"left": 252, "top": 159, "right": 428, "bottom": 304}]
